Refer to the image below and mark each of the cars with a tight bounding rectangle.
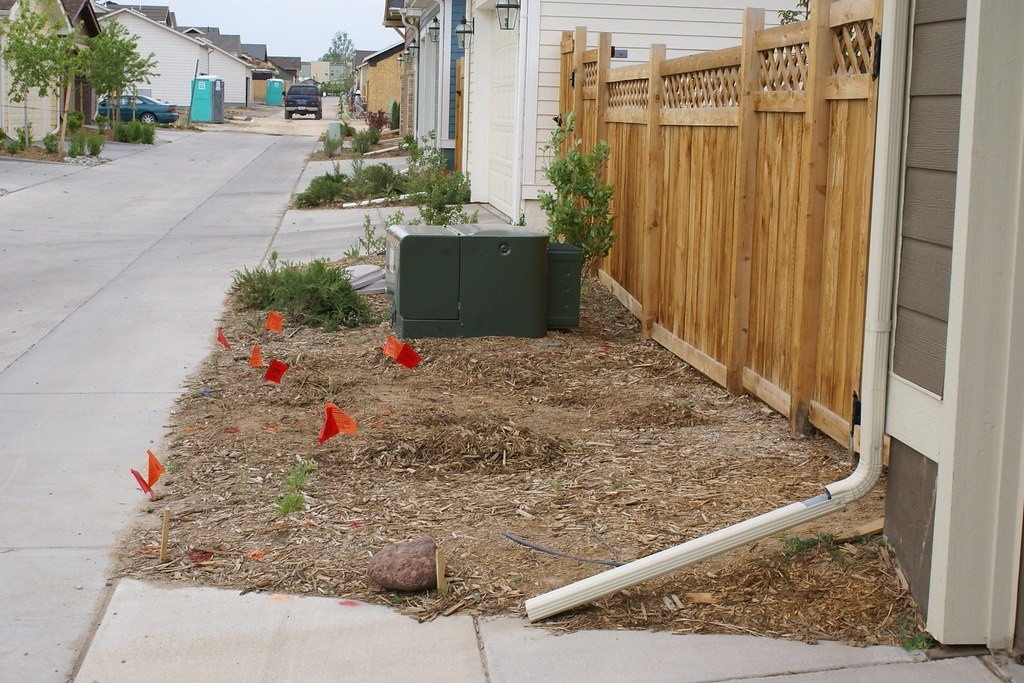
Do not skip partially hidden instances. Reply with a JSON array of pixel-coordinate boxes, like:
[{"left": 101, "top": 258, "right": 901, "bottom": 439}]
[{"left": 97, "top": 94, "right": 180, "bottom": 126}]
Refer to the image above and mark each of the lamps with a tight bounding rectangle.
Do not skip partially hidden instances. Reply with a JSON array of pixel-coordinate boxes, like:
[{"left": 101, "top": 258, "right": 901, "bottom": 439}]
[
  {"left": 403, "top": 49, "right": 415, "bottom": 63},
  {"left": 396, "top": 53, "right": 405, "bottom": 68},
  {"left": 428, "top": 16, "right": 440, "bottom": 42},
  {"left": 406, "top": 38, "right": 419, "bottom": 57},
  {"left": 454, "top": 15, "right": 474, "bottom": 49},
  {"left": 494, "top": 0, "right": 520, "bottom": 30}
]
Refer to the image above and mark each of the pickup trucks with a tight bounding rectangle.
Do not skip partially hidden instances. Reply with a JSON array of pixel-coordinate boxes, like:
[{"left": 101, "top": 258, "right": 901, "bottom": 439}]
[{"left": 281, "top": 84, "right": 327, "bottom": 120}]
